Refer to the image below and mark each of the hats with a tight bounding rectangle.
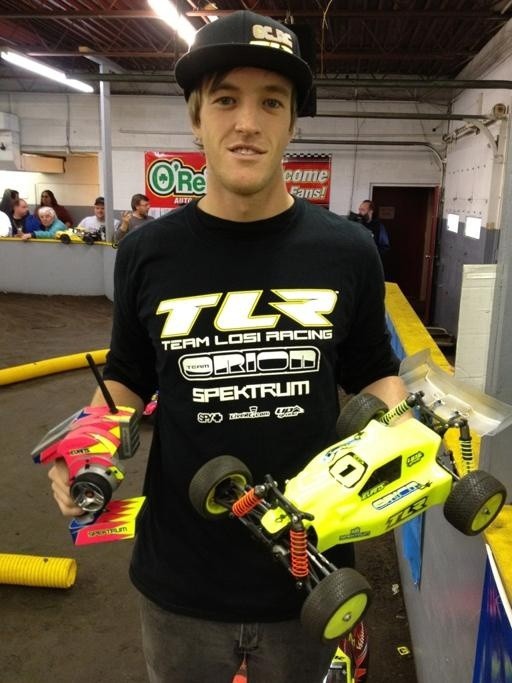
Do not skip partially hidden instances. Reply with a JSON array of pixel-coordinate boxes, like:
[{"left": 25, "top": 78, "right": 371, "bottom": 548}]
[
  {"left": 94, "top": 197, "right": 104, "bottom": 205},
  {"left": 174, "top": 11, "right": 320, "bottom": 121}
]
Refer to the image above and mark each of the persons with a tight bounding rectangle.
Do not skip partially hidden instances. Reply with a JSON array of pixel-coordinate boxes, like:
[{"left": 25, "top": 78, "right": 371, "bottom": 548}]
[
  {"left": 0, "top": 189, "right": 19, "bottom": 239},
  {"left": 21, "top": 204, "right": 71, "bottom": 242},
  {"left": 74, "top": 196, "right": 124, "bottom": 242},
  {"left": 9, "top": 198, "right": 42, "bottom": 237},
  {"left": 348, "top": 197, "right": 394, "bottom": 260},
  {"left": 116, "top": 191, "right": 156, "bottom": 240},
  {"left": 38, "top": 188, "right": 74, "bottom": 228},
  {"left": 44, "top": 10, "right": 419, "bottom": 682}
]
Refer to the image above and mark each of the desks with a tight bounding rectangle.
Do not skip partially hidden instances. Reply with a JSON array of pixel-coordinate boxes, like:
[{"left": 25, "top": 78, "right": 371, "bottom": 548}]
[{"left": 1, "top": 233, "right": 117, "bottom": 302}]
